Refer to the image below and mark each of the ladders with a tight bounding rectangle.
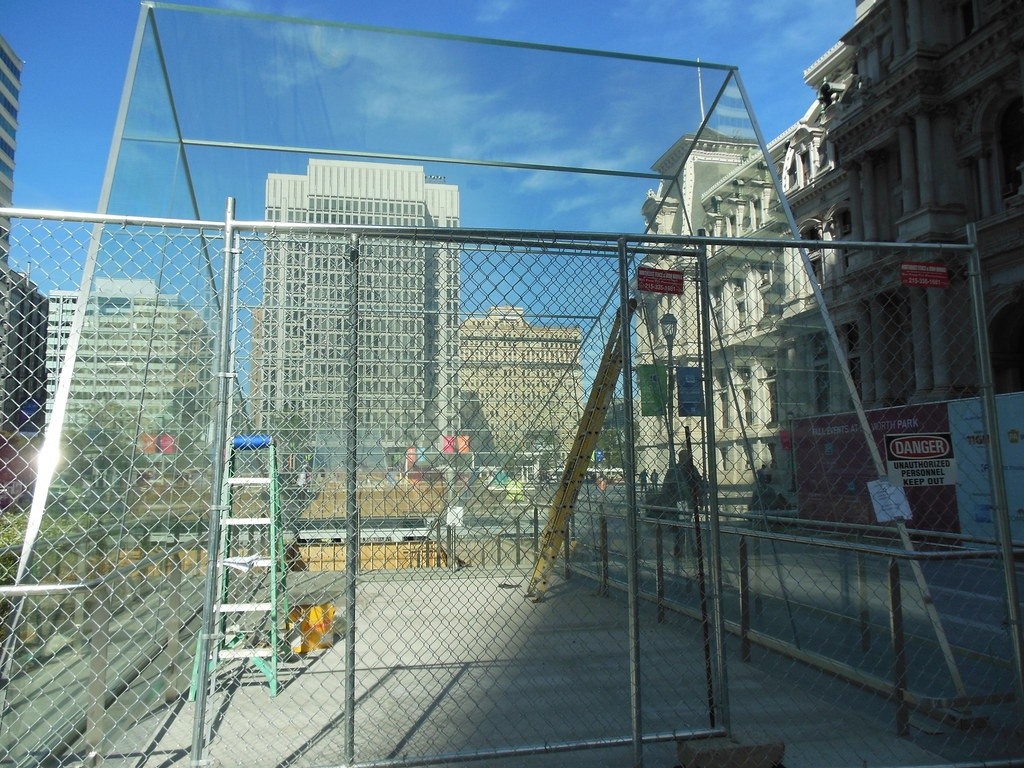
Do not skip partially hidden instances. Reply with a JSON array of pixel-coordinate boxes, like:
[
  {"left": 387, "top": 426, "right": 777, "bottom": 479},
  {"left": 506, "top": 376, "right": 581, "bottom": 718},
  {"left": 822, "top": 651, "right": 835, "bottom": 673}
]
[
  {"left": 523, "top": 297, "right": 637, "bottom": 602},
  {"left": 189, "top": 432, "right": 283, "bottom": 706}
]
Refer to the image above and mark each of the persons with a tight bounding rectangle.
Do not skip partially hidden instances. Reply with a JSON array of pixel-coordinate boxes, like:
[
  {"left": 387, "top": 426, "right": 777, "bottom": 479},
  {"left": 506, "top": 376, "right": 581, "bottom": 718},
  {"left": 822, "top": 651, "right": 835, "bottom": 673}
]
[
  {"left": 638, "top": 468, "right": 649, "bottom": 494},
  {"left": 663, "top": 451, "right": 707, "bottom": 559},
  {"left": 757, "top": 464, "right": 766, "bottom": 479},
  {"left": 297, "top": 461, "right": 308, "bottom": 510},
  {"left": 650, "top": 469, "right": 659, "bottom": 490},
  {"left": 597, "top": 470, "right": 608, "bottom": 500}
]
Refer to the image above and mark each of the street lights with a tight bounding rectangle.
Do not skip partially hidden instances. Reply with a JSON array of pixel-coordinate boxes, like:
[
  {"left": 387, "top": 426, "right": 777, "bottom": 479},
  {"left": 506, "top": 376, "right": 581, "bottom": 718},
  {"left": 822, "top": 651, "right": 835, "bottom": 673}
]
[{"left": 660, "top": 311, "right": 677, "bottom": 469}]
